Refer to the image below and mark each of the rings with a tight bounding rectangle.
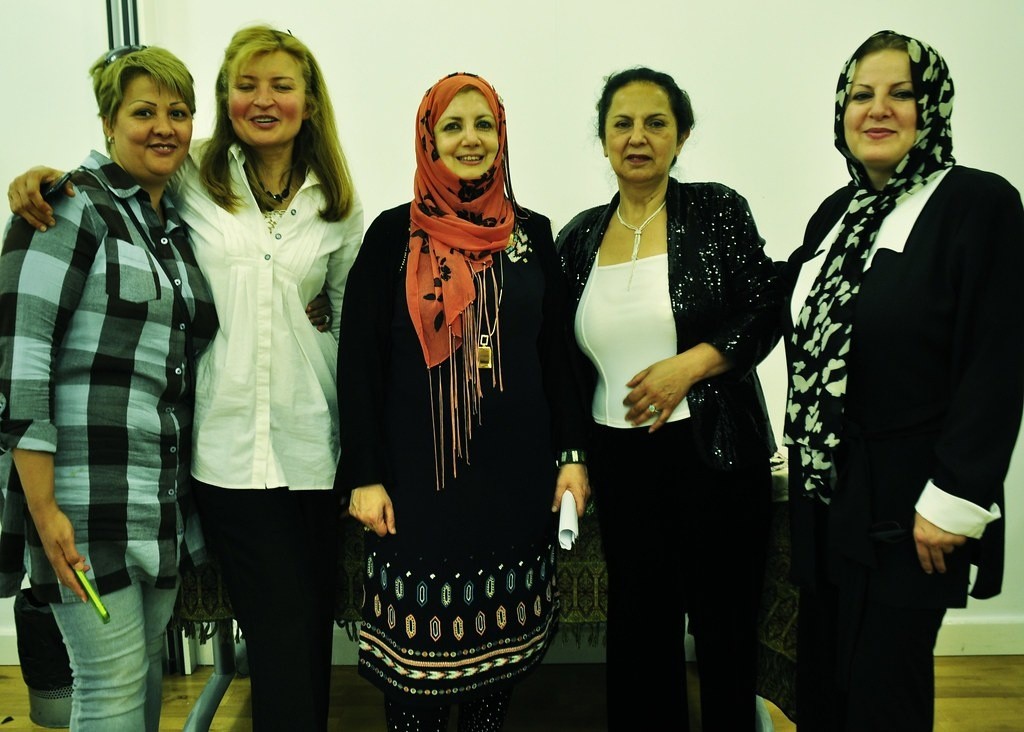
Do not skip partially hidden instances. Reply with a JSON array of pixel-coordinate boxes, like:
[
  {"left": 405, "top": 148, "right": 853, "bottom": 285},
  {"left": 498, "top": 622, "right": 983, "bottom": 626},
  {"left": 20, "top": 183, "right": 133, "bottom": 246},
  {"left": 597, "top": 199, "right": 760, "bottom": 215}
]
[
  {"left": 324, "top": 315, "right": 330, "bottom": 323},
  {"left": 648, "top": 404, "right": 661, "bottom": 415},
  {"left": 363, "top": 526, "right": 374, "bottom": 532}
]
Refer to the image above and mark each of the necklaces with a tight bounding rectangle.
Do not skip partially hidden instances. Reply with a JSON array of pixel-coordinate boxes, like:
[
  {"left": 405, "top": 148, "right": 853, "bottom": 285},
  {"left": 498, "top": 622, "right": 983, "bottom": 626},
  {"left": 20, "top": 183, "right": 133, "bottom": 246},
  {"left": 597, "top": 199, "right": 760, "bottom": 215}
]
[
  {"left": 254, "top": 153, "right": 295, "bottom": 203},
  {"left": 471, "top": 250, "right": 504, "bottom": 369},
  {"left": 155, "top": 206, "right": 159, "bottom": 215},
  {"left": 617, "top": 200, "right": 666, "bottom": 289}
]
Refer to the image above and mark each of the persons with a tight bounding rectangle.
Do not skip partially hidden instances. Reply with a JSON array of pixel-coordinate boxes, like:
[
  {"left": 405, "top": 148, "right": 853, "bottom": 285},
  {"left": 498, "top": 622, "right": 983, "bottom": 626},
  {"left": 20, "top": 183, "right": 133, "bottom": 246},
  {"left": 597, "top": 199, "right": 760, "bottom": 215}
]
[
  {"left": 0, "top": 46, "right": 332, "bottom": 732},
  {"left": 553, "top": 68, "right": 781, "bottom": 732},
  {"left": 5, "top": 26, "right": 366, "bottom": 732},
  {"left": 770, "top": 30, "right": 1024, "bottom": 731},
  {"left": 336, "top": 71, "right": 593, "bottom": 732}
]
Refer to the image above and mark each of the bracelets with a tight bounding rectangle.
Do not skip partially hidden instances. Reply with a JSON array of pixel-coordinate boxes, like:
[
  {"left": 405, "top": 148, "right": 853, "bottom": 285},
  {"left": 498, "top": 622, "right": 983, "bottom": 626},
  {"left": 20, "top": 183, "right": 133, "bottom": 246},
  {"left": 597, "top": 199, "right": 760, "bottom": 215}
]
[{"left": 555, "top": 448, "right": 589, "bottom": 469}]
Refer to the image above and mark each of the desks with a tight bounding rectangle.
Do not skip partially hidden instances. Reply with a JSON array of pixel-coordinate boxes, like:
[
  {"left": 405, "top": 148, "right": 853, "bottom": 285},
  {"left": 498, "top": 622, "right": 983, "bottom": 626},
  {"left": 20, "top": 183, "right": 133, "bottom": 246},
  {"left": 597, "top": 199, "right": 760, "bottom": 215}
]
[{"left": 171, "top": 448, "right": 803, "bottom": 732}]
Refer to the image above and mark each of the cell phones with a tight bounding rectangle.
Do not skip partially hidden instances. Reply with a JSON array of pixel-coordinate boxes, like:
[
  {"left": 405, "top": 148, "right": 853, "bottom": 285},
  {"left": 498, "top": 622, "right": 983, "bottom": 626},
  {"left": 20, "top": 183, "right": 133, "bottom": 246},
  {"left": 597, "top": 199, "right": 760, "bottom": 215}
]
[{"left": 72, "top": 566, "right": 110, "bottom": 624}]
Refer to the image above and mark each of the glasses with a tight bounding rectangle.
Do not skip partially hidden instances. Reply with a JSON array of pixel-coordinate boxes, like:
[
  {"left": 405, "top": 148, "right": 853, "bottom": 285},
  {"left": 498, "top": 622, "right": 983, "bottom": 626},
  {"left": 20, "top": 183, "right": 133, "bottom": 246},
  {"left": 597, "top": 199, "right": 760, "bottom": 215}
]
[{"left": 103, "top": 45, "right": 147, "bottom": 68}]
